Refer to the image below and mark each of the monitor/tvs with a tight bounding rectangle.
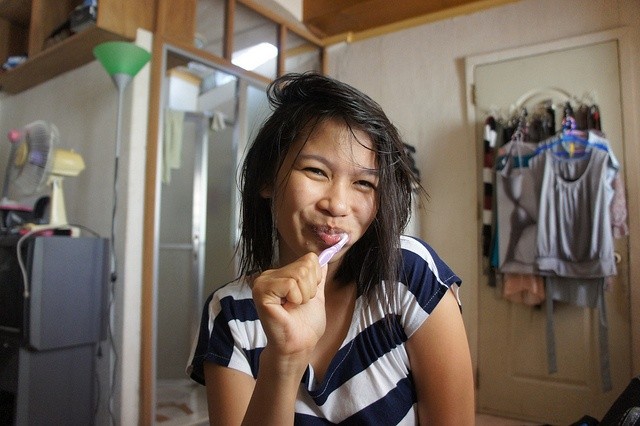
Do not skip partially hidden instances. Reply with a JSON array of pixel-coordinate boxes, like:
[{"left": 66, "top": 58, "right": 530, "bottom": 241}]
[
  {"left": 3, "top": 348, "right": 100, "bottom": 426},
  {"left": 0, "top": 231, "right": 109, "bottom": 353}
]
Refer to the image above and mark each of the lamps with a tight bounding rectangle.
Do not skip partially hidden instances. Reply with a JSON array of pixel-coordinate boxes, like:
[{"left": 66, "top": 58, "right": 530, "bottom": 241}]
[{"left": 92, "top": 40, "right": 152, "bottom": 424}]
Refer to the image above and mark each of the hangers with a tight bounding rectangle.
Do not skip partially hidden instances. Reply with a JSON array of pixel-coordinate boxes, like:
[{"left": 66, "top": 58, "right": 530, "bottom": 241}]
[{"left": 493, "top": 103, "right": 609, "bottom": 171}]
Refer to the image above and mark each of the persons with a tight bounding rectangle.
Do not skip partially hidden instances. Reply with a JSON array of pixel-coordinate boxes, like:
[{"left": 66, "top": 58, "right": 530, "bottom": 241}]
[{"left": 187, "top": 71, "right": 476, "bottom": 425}]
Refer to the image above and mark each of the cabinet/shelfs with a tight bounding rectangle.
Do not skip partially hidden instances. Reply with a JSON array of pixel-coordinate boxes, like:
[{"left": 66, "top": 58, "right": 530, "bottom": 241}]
[{"left": 0, "top": 1, "right": 154, "bottom": 97}]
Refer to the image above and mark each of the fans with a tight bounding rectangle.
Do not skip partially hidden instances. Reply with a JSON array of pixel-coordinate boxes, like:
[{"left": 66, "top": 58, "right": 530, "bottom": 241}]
[{"left": 5, "top": 117, "right": 85, "bottom": 298}]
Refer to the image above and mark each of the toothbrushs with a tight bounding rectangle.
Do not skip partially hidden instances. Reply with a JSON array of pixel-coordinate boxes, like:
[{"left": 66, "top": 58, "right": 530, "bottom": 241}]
[{"left": 307, "top": 232, "right": 348, "bottom": 272}]
